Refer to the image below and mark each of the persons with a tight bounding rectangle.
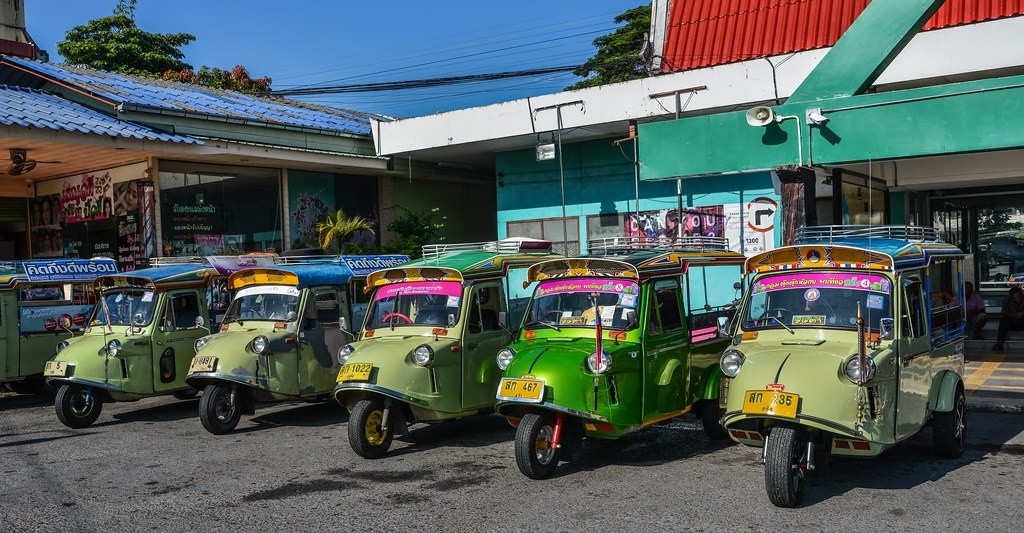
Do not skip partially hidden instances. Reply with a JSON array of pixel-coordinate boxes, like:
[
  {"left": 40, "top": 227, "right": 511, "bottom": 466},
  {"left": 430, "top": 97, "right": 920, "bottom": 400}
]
[
  {"left": 684, "top": 210, "right": 703, "bottom": 237},
  {"left": 120, "top": 180, "right": 138, "bottom": 215},
  {"left": 30, "top": 197, "right": 63, "bottom": 257},
  {"left": 103, "top": 197, "right": 112, "bottom": 218},
  {"left": 993, "top": 286, "right": 1024, "bottom": 351},
  {"left": 666, "top": 211, "right": 678, "bottom": 240},
  {"left": 624, "top": 214, "right": 644, "bottom": 242},
  {"left": 952, "top": 281, "right": 988, "bottom": 340}
]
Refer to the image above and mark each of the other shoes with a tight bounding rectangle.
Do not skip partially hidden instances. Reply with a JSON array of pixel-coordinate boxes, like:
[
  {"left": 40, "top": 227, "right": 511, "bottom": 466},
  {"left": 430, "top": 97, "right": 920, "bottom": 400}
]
[
  {"left": 971, "top": 334, "right": 985, "bottom": 340},
  {"left": 992, "top": 343, "right": 1003, "bottom": 350}
]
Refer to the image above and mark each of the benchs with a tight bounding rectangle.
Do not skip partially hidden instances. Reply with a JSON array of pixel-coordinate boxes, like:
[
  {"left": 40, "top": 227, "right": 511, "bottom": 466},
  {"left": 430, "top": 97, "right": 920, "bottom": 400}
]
[
  {"left": 414, "top": 307, "right": 495, "bottom": 332},
  {"left": 288, "top": 301, "right": 340, "bottom": 322},
  {"left": 789, "top": 286, "right": 955, "bottom": 337},
  {"left": 584, "top": 292, "right": 680, "bottom": 335}
]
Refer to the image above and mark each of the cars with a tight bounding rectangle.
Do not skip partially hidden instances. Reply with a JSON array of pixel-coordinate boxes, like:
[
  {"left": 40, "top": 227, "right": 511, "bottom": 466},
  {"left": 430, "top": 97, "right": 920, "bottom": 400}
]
[{"left": 1008, "top": 273, "right": 1024, "bottom": 290}]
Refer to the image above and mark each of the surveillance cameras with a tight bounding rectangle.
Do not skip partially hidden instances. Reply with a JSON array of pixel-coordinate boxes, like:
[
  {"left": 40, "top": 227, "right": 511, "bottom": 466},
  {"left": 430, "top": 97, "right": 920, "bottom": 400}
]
[{"left": 808, "top": 113, "right": 830, "bottom": 126}]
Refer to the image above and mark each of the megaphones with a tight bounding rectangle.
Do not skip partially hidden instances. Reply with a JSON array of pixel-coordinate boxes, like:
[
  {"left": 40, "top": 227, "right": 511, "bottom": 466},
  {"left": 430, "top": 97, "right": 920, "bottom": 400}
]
[{"left": 746, "top": 106, "right": 773, "bottom": 127}]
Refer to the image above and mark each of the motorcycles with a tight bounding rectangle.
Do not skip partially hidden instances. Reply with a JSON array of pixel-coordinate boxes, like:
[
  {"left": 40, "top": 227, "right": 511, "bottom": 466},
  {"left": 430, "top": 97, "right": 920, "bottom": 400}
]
[
  {"left": 183, "top": 255, "right": 410, "bottom": 436},
  {"left": 716, "top": 224, "right": 970, "bottom": 508},
  {"left": 493, "top": 236, "right": 749, "bottom": 481},
  {"left": 0, "top": 256, "right": 125, "bottom": 393},
  {"left": 44, "top": 251, "right": 288, "bottom": 429},
  {"left": 333, "top": 236, "right": 565, "bottom": 458}
]
